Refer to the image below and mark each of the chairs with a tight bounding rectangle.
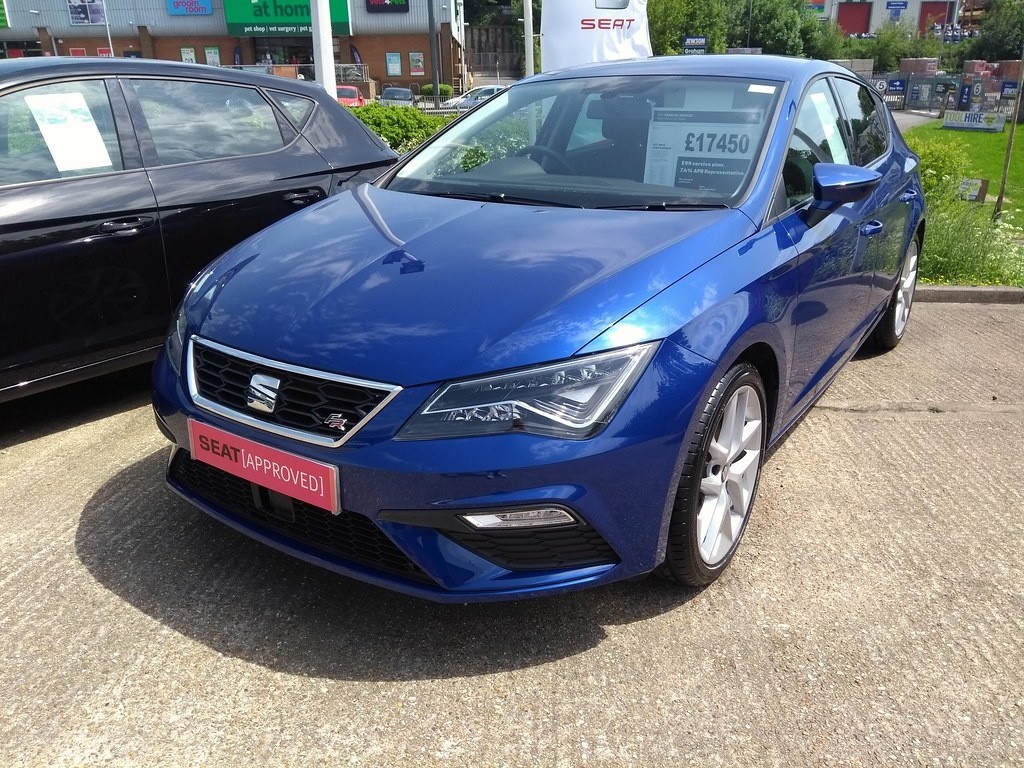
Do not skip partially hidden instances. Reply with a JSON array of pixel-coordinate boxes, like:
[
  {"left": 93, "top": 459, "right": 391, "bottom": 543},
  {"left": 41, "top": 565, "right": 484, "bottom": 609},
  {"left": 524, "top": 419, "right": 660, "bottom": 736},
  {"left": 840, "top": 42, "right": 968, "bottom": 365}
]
[{"left": 575, "top": 98, "right": 653, "bottom": 184}]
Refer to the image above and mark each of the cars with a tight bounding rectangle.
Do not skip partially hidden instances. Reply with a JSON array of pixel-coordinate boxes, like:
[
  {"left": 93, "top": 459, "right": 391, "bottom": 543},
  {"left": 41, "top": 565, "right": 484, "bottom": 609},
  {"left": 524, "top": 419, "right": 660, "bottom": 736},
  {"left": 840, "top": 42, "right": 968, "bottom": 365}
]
[
  {"left": 150, "top": 54, "right": 928, "bottom": 607},
  {"left": 335, "top": 86, "right": 365, "bottom": 107},
  {"left": 456, "top": 87, "right": 507, "bottom": 111},
  {"left": 374, "top": 88, "right": 418, "bottom": 114},
  {"left": 442, "top": 85, "right": 502, "bottom": 109},
  {"left": 0, "top": 58, "right": 400, "bottom": 410}
]
